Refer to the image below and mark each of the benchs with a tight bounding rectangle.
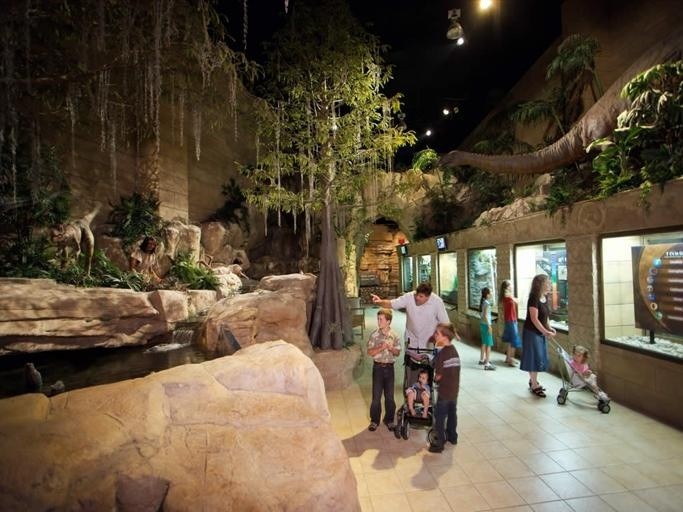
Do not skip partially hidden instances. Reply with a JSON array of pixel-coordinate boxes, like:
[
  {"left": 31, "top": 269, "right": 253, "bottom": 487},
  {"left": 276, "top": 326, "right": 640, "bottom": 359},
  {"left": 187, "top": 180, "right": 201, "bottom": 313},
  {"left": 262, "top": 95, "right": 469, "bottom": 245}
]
[{"left": 347, "top": 307, "right": 368, "bottom": 340}]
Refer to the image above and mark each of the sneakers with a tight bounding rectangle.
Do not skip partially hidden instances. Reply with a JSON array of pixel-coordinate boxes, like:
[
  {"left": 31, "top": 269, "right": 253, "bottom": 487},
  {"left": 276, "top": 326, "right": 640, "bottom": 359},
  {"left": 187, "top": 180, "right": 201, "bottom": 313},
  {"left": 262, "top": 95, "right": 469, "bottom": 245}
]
[
  {"left": 388, "top": 423, "right": 395, "bottom": 431},
  {"left": 479, "top": 359, "right": 518, "bottom": 370},
  {"left": 368, "top": 423, "right": 377, "bottom": 431},
  {"left": 451, "top": 441, "right": 457, "bottom": 445}
]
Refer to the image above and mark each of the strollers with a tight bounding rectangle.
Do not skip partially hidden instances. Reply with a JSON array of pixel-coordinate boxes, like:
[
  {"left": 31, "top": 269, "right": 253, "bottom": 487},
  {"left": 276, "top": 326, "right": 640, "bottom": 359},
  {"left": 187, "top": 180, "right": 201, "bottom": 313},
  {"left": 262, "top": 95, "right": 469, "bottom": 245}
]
[
  {"left": 393, "top": 337, "right": 449, "bottom": 448},
  {"left": 543, "top": 333, "right": 611, "bottom": 416}
]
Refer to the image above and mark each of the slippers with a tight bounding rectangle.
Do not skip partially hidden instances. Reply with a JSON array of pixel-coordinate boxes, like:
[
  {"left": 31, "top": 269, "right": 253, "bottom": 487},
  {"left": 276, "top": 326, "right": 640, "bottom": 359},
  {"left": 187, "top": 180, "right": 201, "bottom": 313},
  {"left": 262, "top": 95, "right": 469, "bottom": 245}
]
[{"left": 529, "top": 380, "right": 547, "bottom": 398}]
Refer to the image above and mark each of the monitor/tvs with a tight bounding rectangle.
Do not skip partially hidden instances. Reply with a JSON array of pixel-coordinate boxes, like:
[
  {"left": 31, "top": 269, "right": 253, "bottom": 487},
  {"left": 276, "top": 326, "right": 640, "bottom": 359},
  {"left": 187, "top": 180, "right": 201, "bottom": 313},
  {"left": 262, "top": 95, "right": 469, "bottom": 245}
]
[
  {"left": 435, "top": 235, "right": 448, "bottom": 251},
  {"left": 400, "top": 245, "right": 407, "bottom": 256}
]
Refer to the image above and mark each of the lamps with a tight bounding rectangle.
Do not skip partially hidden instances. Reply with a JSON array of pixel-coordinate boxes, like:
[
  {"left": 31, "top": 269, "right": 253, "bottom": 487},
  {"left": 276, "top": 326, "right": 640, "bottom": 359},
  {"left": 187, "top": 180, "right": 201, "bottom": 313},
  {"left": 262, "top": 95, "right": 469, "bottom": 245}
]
[{"left": 446, "top": 7, "right": 465, "bottom": 41}]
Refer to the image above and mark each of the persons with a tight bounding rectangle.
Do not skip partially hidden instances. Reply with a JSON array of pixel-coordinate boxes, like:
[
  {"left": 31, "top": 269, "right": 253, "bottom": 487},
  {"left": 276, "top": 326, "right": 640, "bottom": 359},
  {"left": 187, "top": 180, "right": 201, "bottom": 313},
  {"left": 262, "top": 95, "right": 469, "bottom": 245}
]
[
  {"left": 406, "top": 370, "right": 431, "bottom": 418},
  {"left": 520, "top": 274, "right": 556, "bottom": 398},
  {"left": 478, "top": 286, "right": 497, "bottom": 370},
  {"left": 428, "top": 323, "right": 461, "bottom": 454},
  {"left": 498, "top": 280, "right": 523, "bottom": 368},
  {"left": 365, "top": 307, "right": 401, "bottom": 433},
  {"left": 369, "top": 281, "right": 459, "bottom": 356},
  {"left": 128, "top": 235, "right": 163, "bottom": 285},
  {"left": 568, "top": 343, "right": 602, "bottom": 395}
]
